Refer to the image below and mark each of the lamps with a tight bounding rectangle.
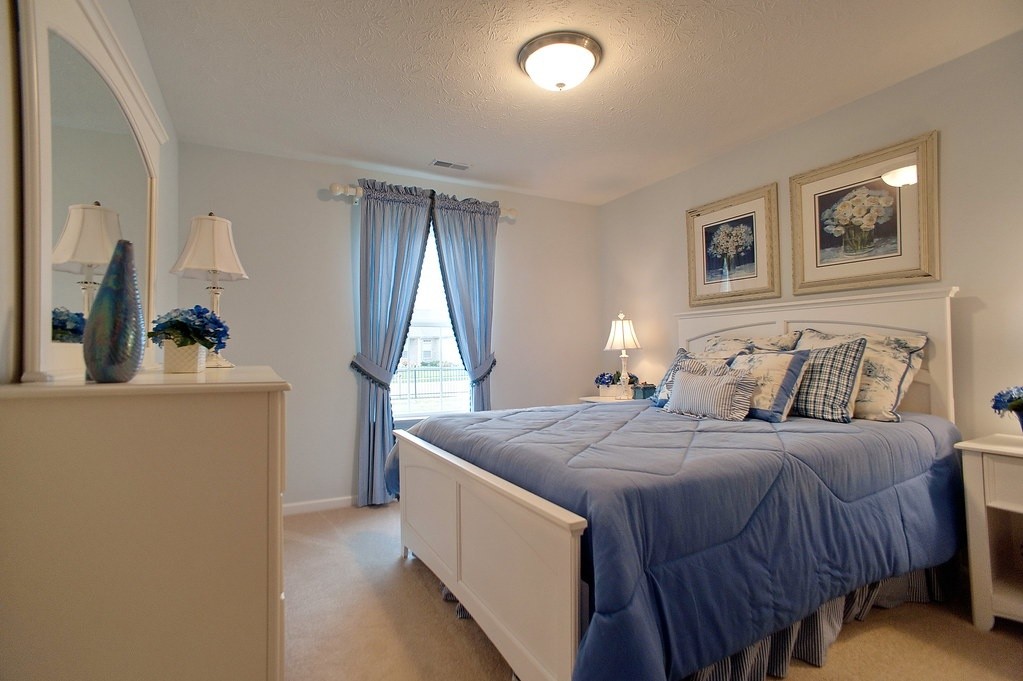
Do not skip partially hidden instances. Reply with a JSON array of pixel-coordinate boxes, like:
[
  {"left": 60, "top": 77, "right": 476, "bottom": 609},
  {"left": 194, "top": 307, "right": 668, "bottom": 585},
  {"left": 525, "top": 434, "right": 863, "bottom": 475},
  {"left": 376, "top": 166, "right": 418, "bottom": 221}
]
[
  {"left": 603, "top": 310, "right": 643, "bottom": 401},
  {"left": 169, "top": 211, "right": 250, "bottom": 368},
  {"left": 518, "top": 32, "right": 601, "bottom": 93},
  {"left": 881, "top": 164, "right": 919, "bottom": 187},
  {"left": 49, "top": 200, "right": 122, "bottom": 317}
]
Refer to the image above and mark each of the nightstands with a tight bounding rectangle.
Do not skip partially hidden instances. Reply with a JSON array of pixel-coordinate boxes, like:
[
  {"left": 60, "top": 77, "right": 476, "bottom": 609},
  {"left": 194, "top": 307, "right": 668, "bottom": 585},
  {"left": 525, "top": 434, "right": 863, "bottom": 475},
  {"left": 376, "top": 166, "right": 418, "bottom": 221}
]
[
  {"left": 579, "top": 397, "right": 634, "bottom": 403},
  {"left": 953, "top": 434, "right": 1023, "bottom": 632}
]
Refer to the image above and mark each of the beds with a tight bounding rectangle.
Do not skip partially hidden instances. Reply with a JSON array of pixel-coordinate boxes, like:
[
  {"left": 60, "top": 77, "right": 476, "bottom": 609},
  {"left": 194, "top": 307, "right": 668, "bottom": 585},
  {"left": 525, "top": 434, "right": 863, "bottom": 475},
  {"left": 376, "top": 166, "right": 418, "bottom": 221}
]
[{"left": 392, "top": 287, "right": 963, "bottom": 681}]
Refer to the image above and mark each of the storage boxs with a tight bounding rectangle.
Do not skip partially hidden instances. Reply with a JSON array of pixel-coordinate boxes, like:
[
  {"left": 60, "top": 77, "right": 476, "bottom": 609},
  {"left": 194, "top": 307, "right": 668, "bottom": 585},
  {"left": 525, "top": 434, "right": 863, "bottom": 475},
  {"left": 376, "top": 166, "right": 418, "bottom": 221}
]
[{"left": 632, "top": 384, "right": 656, "bottom": 400}]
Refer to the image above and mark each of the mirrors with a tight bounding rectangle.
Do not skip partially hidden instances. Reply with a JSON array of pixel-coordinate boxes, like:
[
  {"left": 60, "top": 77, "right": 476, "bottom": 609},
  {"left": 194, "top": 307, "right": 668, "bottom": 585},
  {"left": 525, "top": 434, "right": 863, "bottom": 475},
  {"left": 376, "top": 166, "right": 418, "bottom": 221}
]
[{"left": 45, "top": 20, "right": 157, "bottom": 351}]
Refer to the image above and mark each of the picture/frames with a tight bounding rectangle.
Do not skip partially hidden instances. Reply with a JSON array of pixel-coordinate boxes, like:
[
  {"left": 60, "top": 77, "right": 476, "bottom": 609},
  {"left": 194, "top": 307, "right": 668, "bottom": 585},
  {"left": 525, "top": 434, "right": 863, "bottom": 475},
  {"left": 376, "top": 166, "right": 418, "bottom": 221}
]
[
  {"left": 686, "top": 182, "right": 780, "bottom": 307},
  {"left": 793, "top": 130, "right": 939, "bottom": 296}
]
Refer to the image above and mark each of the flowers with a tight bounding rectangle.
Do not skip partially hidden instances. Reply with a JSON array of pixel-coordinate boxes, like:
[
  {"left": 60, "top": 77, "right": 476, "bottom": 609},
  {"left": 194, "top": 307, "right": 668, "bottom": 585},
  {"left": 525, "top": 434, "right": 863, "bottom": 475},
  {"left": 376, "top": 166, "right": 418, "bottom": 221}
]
[
  {"left": 51, "top": 304, "right": 86, "bottom": 343},
  {"left": 706, "top": 224, "right": 754, "bottom": 262},
  {"left": 147, "top": 306, "right": 230, "bottom": 355},
  {"left": 595, "top": 372, "right": 638, "bottom": 389},
  {"left": 821, "top": 186, "right": 894, "bottom": 236},
  {"left": 991, "top": 386, "right": 1023, "bottom": 434}
]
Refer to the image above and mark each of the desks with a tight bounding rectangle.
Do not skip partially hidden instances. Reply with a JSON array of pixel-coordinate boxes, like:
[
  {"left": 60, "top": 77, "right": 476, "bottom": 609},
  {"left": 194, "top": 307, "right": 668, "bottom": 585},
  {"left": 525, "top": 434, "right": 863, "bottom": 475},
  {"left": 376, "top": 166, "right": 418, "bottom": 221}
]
[{"left": 3, "top": 361, "right": 293, "bottom": 680}]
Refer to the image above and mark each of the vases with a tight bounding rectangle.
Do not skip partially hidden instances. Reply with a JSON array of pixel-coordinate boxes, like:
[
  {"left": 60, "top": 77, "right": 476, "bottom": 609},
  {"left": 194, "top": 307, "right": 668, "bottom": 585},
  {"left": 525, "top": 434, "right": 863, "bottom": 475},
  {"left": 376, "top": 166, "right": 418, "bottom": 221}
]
[
  {"left": 163, "top": 338, "right": 205, "bottom": 373},
  {"left": 842, "top": 226, "right": 875, "bottom": 255},
  {"left": 723, "top": 257, "right": 735, "bottom": 276},
  {"left": 82, "top": 239, "right": 146, "bottom": 384}
]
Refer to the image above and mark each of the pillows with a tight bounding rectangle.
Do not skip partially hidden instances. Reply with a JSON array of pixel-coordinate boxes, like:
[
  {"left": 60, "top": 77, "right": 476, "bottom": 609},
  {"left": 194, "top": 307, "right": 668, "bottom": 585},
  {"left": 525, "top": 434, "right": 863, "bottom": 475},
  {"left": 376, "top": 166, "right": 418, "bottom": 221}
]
[
  {"left": 696, "top": 328, "right": 928, "bottom": 424},
  {"left": 651, "top": 347, "right": 744, "bottom": 407},
  {"left": 661, "top": 359, "right": 757, "bottom": 422}
]
